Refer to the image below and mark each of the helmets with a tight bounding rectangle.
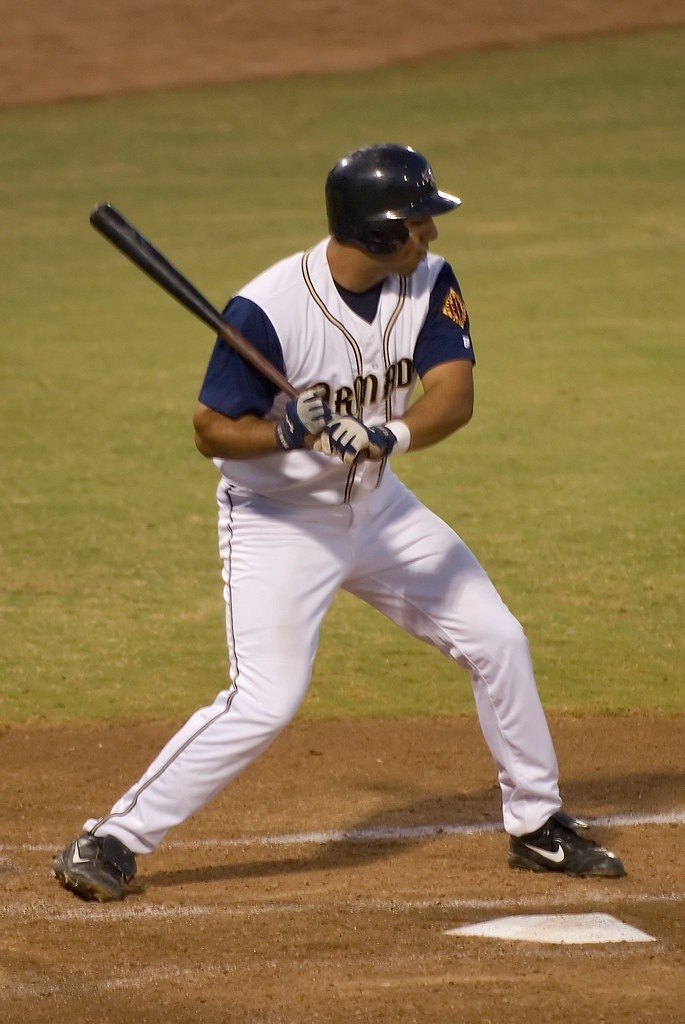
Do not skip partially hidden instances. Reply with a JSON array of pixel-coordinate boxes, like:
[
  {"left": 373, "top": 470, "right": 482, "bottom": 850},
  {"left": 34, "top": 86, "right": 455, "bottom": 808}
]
[{"left": 325, "top": 141, "right": 459, "bottom": 253}]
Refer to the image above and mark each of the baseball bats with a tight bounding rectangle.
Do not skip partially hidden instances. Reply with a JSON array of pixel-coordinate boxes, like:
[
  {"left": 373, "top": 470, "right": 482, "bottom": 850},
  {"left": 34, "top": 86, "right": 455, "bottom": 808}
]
[{"left": 88, "top": 201, "right": 367, "bottom": 464}]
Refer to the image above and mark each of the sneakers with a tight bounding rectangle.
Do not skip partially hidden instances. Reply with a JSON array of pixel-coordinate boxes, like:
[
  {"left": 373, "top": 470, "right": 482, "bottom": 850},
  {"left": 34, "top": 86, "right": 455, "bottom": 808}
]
[
  {"left": 51, "top": 833, "right": 140, "bottom": 904},
  {"left": 509, "top": 812, "right": 624, "bottom": 880}
]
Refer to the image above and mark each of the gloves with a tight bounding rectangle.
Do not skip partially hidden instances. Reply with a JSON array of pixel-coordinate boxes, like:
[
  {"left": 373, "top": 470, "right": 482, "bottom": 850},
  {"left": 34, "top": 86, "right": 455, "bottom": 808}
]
[{"left": 275, "top": 389, "right": 399, "bottom": 467}]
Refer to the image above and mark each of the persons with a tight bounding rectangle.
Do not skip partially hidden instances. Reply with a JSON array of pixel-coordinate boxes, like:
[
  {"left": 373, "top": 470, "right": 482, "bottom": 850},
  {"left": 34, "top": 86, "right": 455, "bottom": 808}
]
[{"left": 49, "top": 142, "right": 625, "bottom": 904}]
